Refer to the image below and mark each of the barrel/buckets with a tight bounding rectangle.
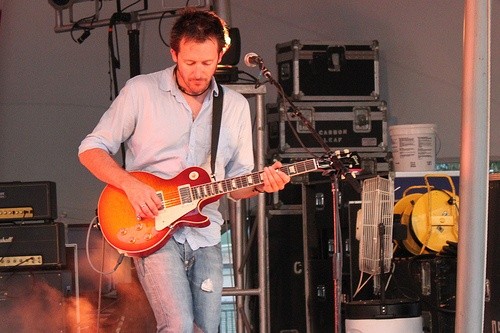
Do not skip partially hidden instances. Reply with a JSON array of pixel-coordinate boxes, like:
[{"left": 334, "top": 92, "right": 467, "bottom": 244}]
[{"left": 389, "top": 124, "right": 441, "bottom": 172}]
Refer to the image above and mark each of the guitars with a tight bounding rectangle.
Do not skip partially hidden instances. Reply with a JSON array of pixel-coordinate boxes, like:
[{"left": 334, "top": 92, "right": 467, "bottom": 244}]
[{"left": 96, "top": 148, "right": 363, "bottom": 258}]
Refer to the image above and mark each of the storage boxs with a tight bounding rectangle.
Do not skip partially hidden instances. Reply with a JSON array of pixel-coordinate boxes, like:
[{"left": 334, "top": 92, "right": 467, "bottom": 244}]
[
  {"left": 276, "top": 39, "right": 381, "bottom": 101},
  {"left": 265, "top": 154, "right": 378, "bottom": 210},
  {"left": 265, "top": 101, "right": 389, "bottom": 153}
]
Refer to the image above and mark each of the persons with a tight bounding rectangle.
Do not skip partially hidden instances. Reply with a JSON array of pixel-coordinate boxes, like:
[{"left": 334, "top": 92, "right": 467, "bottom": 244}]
[{"left": 78, "top": 10, "right": 291, "bottom": 333}]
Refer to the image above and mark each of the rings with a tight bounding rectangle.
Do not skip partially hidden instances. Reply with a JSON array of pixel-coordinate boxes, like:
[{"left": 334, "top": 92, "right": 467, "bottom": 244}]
[{"left": 141, "top": 205, "right": 147, "bottom": 208}]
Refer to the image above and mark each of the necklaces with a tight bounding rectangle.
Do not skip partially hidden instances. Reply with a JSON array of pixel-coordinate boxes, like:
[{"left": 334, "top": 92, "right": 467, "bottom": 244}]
[{"left": 175, "top": 68, "right": 212, "bottom": 96}]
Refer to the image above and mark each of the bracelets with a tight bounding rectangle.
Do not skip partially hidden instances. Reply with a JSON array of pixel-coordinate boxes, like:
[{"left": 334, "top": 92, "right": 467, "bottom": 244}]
[{"left": 253, "top": 187, "right": 266, "bottom": 193}]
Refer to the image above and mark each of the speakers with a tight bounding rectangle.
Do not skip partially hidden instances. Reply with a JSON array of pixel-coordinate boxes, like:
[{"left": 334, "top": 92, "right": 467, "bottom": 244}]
[{"left": 0, "top": 270, "right": 67, "bottom": 333}]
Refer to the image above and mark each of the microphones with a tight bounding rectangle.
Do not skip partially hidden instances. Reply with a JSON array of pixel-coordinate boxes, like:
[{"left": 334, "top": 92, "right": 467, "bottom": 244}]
[{"left": 243, "top": 52, "right": 263, "bottom": 67}]
